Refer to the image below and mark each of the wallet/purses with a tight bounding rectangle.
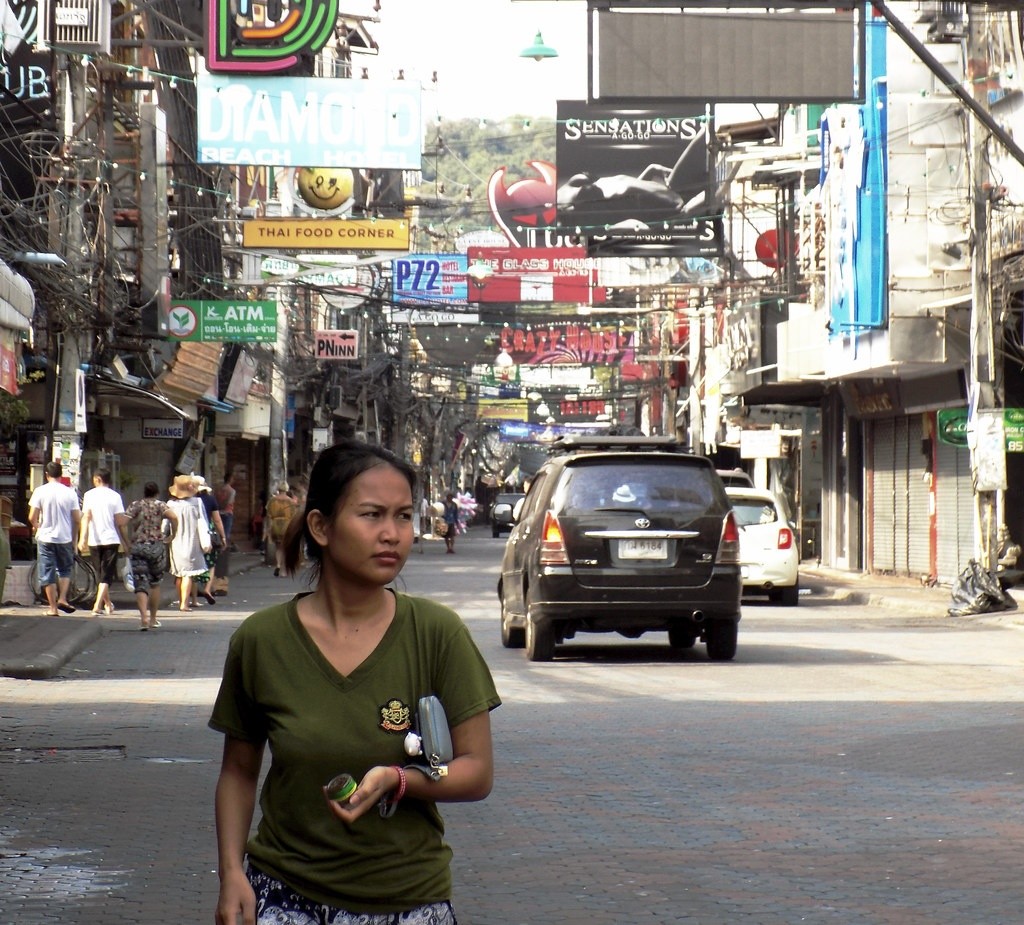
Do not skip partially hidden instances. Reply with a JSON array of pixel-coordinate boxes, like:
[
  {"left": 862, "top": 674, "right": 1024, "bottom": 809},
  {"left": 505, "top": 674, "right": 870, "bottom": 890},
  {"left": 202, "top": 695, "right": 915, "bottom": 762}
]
[{"left": 379, "top": 696, "right": 453, "bottom": 820}]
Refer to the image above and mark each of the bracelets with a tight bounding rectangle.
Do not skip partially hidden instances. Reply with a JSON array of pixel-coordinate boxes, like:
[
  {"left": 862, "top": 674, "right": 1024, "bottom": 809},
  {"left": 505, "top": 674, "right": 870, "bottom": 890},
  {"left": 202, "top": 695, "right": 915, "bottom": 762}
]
[{"left": 379, "top": 764, "right": 405, "bottom": 818}]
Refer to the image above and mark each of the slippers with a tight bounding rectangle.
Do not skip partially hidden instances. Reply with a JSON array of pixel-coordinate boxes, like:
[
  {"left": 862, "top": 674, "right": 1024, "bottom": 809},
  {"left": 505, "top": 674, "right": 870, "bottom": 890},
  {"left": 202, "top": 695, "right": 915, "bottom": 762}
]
[
  {"left": 137, "top": 624, "right": 149, "bottom": 631},
  {"left": 57, "top": 603, "right": 75, "bottom": 613},
  {"left": 150, "top": 620, "right": 161, "bottom": 627},
  {"left": 45, "top": 610, "right": 59, "bottom": 616}
]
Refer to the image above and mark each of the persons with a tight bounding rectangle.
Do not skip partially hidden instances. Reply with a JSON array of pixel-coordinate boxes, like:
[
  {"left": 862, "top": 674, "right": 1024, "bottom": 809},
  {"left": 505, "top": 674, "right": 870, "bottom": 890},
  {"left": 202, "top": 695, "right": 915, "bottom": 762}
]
[
  {"left": 439, "top": 490, "right": 461, "bottom": 552},
  {"left": 117, "top": 481, "right": 180, "bottom": 630},
  {"left": 28, "top": 461, "right": 81, "bottom": 615},
  {"left": 78, "top": 467, "right": 129, "bottom": 615},
  {"left": 186, "top": 476, "right": 227, "bottom": 607},
  {"left": 202, "top": 442, "right": 504, "bottom": 924},
  {"left": 218, "top": 472, "right": 309, "bottom": 576},
  {"left": 161, "top": 473, "right": 208, "bottom": 611}
]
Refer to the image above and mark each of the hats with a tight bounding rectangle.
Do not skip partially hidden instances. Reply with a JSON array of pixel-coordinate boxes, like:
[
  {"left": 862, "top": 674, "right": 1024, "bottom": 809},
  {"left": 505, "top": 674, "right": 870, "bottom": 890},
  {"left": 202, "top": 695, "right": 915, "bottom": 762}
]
[
  {"left": 167, "top": 475, "right": 200, "bottom": 499},
  {"left": 192, "top": 476, "right": 213, "bottom": 494}
]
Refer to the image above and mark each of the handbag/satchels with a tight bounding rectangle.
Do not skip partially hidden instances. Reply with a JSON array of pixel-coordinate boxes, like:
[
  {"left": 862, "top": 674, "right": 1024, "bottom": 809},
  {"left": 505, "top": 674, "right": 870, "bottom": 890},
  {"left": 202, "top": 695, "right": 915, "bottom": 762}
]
[{"left": 120, "top": 557, "right": 135, "bottom": 593}]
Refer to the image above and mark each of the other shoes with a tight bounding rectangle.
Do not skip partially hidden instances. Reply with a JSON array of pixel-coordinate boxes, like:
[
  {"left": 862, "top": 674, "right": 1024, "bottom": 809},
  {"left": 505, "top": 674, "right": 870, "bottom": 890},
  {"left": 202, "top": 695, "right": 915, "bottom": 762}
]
[{"left": 203, "top": 590, "right": 215, "bottom": 605}]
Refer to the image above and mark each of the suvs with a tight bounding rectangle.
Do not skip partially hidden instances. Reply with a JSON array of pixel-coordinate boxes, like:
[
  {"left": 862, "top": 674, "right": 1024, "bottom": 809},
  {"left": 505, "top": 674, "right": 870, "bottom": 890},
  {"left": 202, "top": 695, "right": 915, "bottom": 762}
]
[
  {"left": 493, "top": 451, "right": 743, "bottom": 662},
  {"left": 489, "top": 492, "right": 527, "bottom": 538},
  {"left": 716, "top": 468, "right": 757, "bottom": 489},
  {"left": 722, "top": 486, "right": 800, "bottom": 606}
]
